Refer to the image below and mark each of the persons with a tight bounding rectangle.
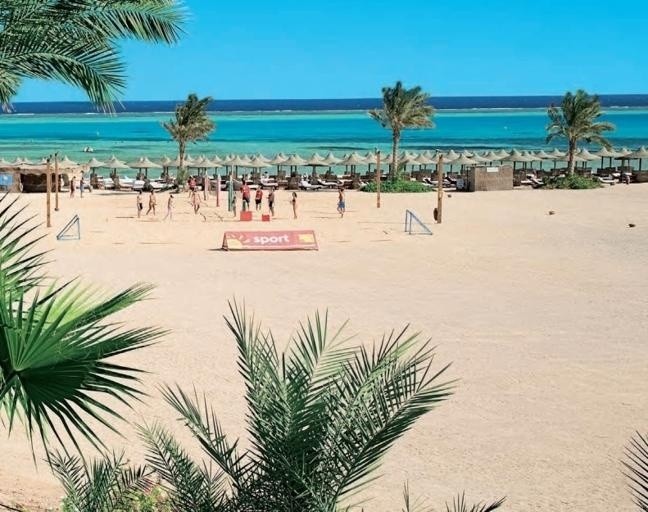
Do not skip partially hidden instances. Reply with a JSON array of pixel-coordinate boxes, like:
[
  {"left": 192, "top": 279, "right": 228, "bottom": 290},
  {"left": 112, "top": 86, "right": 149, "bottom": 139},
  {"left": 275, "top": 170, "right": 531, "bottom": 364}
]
[
  {"left": 80, "top": 176, "right": 87, "bottom": 198},
  {"left": 145, "top": 190, "right": 158, "bottom": 216},
  {"left": 340, "top": 188, "right": 345, "bottom": 214},
  {"left": 255, "top": 185, "right": 263, "bottom": 213},
  {"left": 230, "top": 194, "right": 236, "bottom": 218},
  {"left": 89, "top": 169, "right": 470, "bottom": 198},
  {"left": 69, "top": 175, "right": 77, "bottom": 198},
  {"left": 190, "top": 190, "right": 202, "bottom": 216},
  {"left": 337, "top": 187, "right": 344, "bottom": 218},
  {"left": 162, "top": 194, "right": 176, "bottom": 223},
  {"left": 240, "top": 180, "right": 251, "bottom": 211},
  {"left": 267, "top": 186, "right": 275, "bottom": 217},
  {"left": 137, "top": 189, "right": 144, "bottom": 218},
  {"left": 289, "top": 191, "right": 297, "bottom": 220}
]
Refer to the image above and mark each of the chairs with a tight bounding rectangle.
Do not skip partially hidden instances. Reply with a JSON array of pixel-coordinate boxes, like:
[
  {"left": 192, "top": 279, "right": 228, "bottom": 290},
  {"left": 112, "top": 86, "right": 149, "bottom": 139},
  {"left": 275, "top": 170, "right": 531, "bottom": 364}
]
[{"left": 59, "top": 165, "right": 632, "bottom": 190}]
